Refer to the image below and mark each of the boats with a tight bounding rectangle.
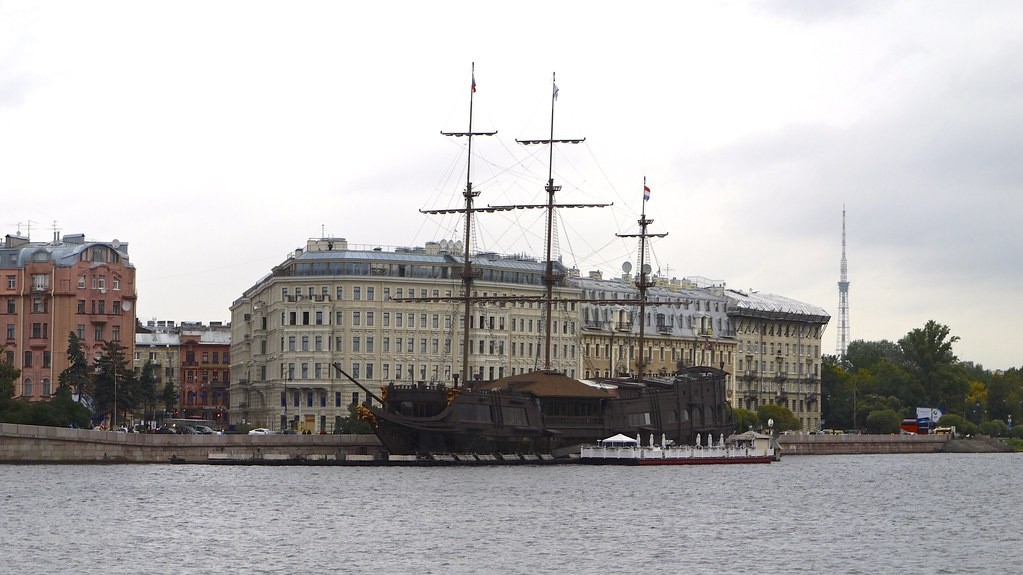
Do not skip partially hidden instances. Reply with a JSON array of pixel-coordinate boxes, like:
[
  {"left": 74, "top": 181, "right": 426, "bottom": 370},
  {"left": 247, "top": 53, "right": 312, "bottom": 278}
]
[{"left": 580, "top": 424, "right": 776, "bottom": 465}]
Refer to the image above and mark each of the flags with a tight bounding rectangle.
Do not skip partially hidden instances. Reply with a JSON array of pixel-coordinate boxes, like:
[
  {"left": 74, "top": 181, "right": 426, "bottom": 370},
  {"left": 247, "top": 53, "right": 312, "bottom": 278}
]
[{"left": 643, "top": 185, "right": 650, "bottom": 201}]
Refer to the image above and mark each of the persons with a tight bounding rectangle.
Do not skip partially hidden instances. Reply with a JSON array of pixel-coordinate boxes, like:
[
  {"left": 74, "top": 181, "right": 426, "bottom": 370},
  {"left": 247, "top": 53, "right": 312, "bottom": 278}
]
[
  {"left": 302, "top": 430, "right": 306, "bottom": 434},
  {"left": 307, "top": 430, "right": 311, "bottom": 434}
]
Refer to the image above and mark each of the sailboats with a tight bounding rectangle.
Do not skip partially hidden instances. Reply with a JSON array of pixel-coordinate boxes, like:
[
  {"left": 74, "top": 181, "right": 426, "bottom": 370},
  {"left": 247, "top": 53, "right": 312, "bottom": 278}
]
[{"left": 333, "top": 59, "right": 737, "bottom": 458}]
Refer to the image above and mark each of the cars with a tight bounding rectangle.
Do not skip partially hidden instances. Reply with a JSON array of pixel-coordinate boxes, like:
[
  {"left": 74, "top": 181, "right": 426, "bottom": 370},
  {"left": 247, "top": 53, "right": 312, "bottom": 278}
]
[
  {"left": 785, "top": 417, "right": 975, "bottom": 448},
  {"left": 89, "top": 409, "right": 288, "bottom": 435}
]
